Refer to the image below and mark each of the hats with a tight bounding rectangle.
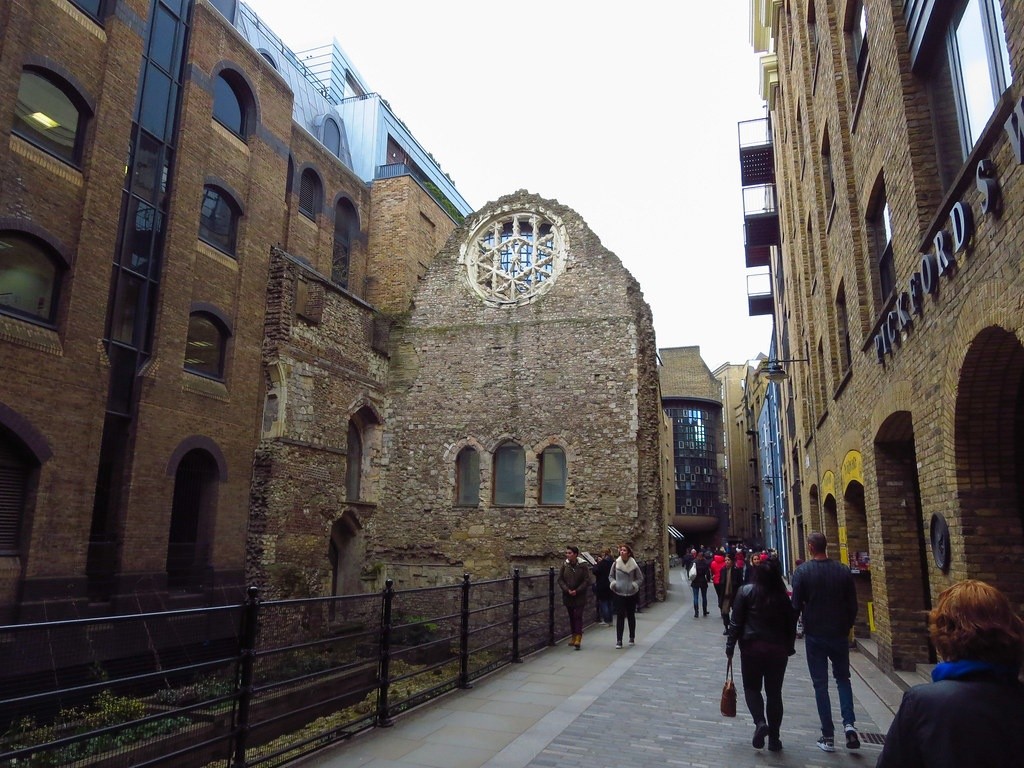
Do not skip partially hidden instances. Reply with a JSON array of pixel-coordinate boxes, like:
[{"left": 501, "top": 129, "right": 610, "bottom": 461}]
[
  {"left": 567, "top": 545, "right": 578, "bottom": 555},
  {"left": 795, "top": 559, "right": 804, "bottom": 566}
]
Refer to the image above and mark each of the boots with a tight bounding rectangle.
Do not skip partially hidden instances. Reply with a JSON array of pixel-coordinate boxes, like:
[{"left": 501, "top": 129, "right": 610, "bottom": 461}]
[
  {"left": 703, "top": 607, "right": 710, "bottom": 616},
  {"left": 568, "top": 633, "right": 575, "bottom": 646},
  {"left": 694, "top": 606, "right": 698, "bottom": 618},
  {"left": 574, "top": 634, "right": 581, "bottom": 649}
]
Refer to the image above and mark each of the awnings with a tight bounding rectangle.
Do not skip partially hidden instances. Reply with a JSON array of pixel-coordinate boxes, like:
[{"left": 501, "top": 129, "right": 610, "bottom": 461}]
[{"left": 668, "top": 526, "right": 684, "bottom": 539}]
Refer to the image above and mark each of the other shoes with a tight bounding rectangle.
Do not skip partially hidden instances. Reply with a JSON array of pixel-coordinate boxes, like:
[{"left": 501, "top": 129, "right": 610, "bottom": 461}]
[
  {"left": 752, "top": 723, "right": 768, "bottom": 749},
  {"left": 723, "top": 628, "right": 728, "bottom": 635},
  {"left": 616, "top": 641, "right": 622, "bottom": 649},
  {"left": 768, "top": 740, "right": 782, "bottom": 751},
  {"left": 798, "top": 633, "right": 802, "bottom": 639},
  {"left": 599, "top": 621, "right": 613, "bottom": 627},
  {"left": 629, "top": 639, "right": 635, "bottom": 645}
]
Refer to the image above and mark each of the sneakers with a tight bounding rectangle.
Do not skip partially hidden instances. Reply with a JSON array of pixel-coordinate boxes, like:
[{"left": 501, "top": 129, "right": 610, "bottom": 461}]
[
  {"left": 844, "top": 724, "right": 860, "bottom": 749},
  {"left": 816, "top": 736, "right": 835, "bottom": 751}
]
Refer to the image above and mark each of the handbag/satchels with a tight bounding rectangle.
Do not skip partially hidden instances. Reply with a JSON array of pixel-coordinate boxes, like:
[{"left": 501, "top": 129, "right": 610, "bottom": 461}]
[
  {"left": 686, "top": 562, "right": 697, "bottom": 582},
  {"left": 720, "top": 659, "right": 737, "bottom": 717}
]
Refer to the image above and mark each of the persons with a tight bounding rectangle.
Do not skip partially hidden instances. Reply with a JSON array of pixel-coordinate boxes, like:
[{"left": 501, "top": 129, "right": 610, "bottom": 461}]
[
  {"left": 791, "top": 533, "right": 861, "bottom": 751},
  {"left": 592, "top": 544, "right": 644, "bottom": 649},
  {"left": 682, "top": 545, "right": 782, "bottom": 635},
  {"left": 557, "top": 545, "right": 588, "bottom": 649},
  {"left": 875, "top": 580, "right": 1024, "bottom": 768},
  {"left": 726, "top": 559, "right": 797, "bottom": 751}
]
